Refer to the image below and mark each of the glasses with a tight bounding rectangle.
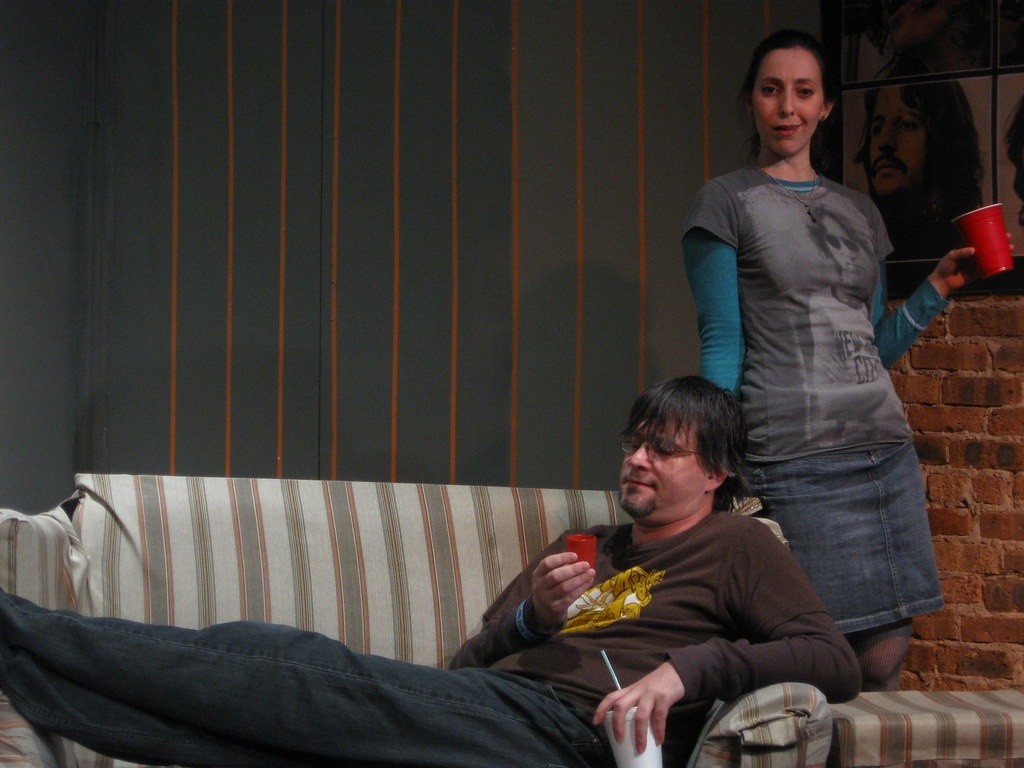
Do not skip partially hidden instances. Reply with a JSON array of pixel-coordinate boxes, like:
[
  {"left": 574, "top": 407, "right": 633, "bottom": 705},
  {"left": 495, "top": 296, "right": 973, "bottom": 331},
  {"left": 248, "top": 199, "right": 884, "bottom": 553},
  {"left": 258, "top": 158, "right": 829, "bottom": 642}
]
[{"left": 620, "top": 428, "right": 698, "bottom": 458}]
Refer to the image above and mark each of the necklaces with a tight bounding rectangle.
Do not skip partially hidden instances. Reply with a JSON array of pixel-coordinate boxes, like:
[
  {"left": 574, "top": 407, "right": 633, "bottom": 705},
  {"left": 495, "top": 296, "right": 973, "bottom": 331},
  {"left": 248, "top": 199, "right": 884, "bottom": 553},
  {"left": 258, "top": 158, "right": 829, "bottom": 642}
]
[{"left": 752, "top": 163, "right": 818, "bottom": 222}]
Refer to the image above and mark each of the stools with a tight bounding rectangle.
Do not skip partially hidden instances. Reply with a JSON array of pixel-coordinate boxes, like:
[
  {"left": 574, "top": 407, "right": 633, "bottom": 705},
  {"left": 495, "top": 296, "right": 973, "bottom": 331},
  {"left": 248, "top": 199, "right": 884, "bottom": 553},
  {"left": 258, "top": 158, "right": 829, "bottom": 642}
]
[{"left": 830, "top": 689, "right": 1024, "bottom": 768}]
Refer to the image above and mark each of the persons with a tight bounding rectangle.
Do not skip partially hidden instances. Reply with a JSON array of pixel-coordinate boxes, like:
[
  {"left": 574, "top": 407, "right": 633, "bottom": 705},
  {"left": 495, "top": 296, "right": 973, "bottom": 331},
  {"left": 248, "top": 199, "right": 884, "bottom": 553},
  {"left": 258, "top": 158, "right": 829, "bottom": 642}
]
[
  {"left": 0, "top": 374, "right": 862, "bottom": 768},
  {"left": 679, "top": 29, "right": 1015, "bottom": 690},
  {"left": 849, "top": 81, "right": 984, "bottom": 264}
]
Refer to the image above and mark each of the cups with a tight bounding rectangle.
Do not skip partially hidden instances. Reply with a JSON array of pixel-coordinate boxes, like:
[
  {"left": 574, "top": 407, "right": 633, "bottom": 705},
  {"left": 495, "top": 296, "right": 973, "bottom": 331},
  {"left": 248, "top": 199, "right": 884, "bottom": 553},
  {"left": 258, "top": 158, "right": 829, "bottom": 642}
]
[
  {"left": 950, "top": 203, "right": 1014, "bottom": 278},
  {"left": 603, "top": 706, "right": 663, "bottom": 768},
  {"left": 567, "top": 534, "right": 597, "bottom": 570}
]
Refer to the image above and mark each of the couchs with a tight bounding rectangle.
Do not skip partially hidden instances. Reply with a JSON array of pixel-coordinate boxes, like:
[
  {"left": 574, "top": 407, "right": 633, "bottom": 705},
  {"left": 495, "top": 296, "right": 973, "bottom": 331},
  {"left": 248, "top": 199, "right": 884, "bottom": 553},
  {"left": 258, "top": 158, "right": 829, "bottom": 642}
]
[{"left": 0, "top": 472, "right": 833, "bottom": 768}]
